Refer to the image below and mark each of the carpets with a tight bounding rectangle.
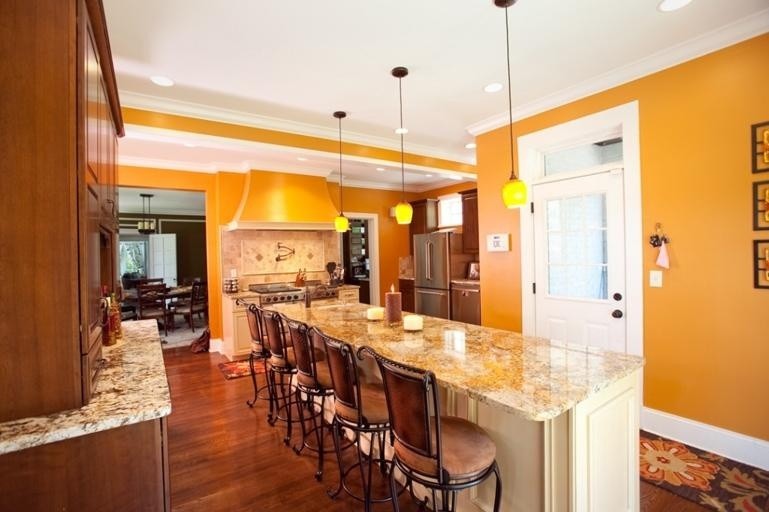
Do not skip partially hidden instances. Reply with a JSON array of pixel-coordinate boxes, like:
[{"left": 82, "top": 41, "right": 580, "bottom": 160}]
[
  {"left": 153, "top": 313, "right": 208, "bottom": 349},
  {"left": 641, "top": 430, "right": 769, "bottom": 512},
  {"left": 216, "top": 359, "right": 268, "bottom": 381}
]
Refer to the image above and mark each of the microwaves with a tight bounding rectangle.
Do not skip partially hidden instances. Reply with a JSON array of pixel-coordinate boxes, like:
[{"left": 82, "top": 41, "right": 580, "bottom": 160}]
[{"left": 353, "top": 265, "right": 369, "bottom": 278}]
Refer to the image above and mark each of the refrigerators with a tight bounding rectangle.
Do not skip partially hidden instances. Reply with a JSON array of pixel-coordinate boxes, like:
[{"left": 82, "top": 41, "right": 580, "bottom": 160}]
[{"left": 412, "top": 232, "right": 463, "bottom": 320}]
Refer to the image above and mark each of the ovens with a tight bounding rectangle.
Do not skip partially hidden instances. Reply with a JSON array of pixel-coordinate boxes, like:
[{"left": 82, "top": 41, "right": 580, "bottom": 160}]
[{"left": 451, "top": 281, "right": 481, "bottom": 326}]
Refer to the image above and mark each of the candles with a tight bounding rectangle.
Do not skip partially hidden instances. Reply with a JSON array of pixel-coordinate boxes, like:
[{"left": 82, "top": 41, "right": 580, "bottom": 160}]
[{"left": 385, "top": 282, "right": 402, "bottom": 323}]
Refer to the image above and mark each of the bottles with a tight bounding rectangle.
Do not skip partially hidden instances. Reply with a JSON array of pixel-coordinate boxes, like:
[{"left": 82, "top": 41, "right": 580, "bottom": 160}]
[
  {"left": 102, "top": 286, "right": 122, "bottom": 346},
  {"left": 294, "top": 268, "right": 307, "bottom": 287}
]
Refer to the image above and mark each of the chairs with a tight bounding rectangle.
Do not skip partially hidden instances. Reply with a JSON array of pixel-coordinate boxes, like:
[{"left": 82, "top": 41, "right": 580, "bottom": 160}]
[{"left": 119, "top": 275, "right": 207, "bottom": 337}]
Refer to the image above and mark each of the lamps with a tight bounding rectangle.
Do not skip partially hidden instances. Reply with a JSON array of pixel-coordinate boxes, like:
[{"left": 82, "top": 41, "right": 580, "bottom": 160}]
[
  {"left": 493, "top": 0, "right": 530, "bottom": 209},
  {"left": 392, "top": 65, "right": 413, "bottom": 225},
  {"left": 137, "top": 193, "right": 155, "bottom": 235},
  {"left": 333, "top": 110, "right": 349, "bottom": 233}
]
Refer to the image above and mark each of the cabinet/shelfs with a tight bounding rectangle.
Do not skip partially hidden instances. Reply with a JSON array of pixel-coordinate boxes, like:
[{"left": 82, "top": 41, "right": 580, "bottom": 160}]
[
  {"left": 0, "top": 0, "right": 123, "bottom": 429},
  {"left": 339, "top": 288, "right": 359, "bottom": 303},
  {"left": 408, "top": 200, "right": 438, "bottom": 255},
  {"left": 460, "top": 191, "right": 479, "bottom": 254},
  {"left": 222, "top": 294, "right": 261, "bottom": 361},
  {"left": 398, "top": 279, "right": 415, "bottom": 313}
]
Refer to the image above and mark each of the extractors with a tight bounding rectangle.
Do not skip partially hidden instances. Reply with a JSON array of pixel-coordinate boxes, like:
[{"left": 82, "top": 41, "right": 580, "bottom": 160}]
[{"left": 226, "top": 223, "right": 341, "bottom": 232}]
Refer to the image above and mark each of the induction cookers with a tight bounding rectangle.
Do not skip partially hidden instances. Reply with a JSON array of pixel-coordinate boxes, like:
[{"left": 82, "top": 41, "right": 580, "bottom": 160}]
[
  {"left": 307, "top": 283, "right": 336, "bottom": 290},
  {"left": 249, "top": 281, "right": 302, "bottom": 293}
]
